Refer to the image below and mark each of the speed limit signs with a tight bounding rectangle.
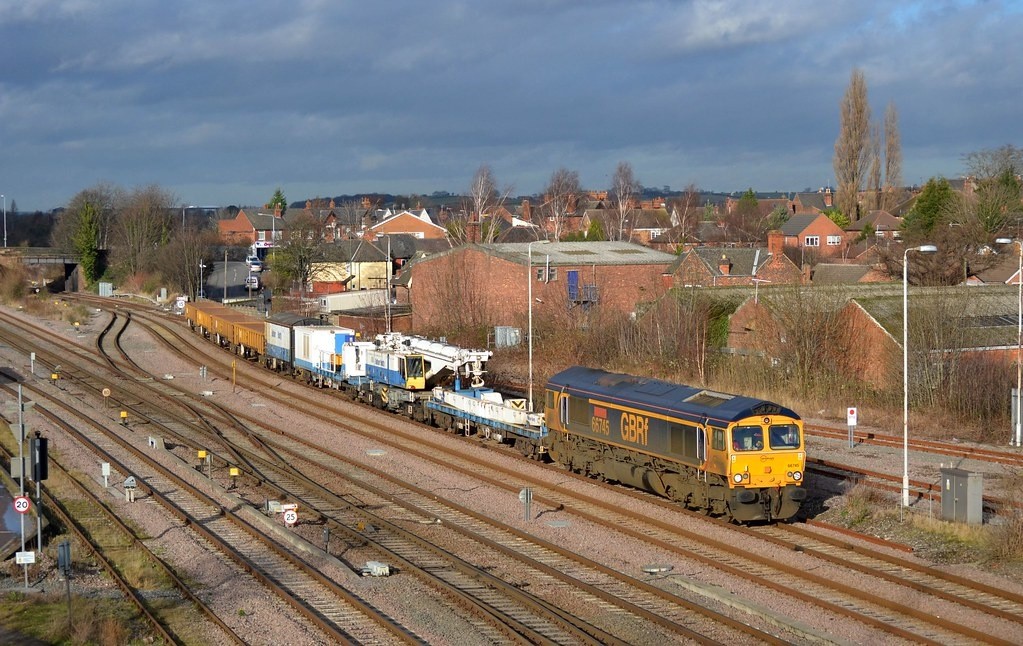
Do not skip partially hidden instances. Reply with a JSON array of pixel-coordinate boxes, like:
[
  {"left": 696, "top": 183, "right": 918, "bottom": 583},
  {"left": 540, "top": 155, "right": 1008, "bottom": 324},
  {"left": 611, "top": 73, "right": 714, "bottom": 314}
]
[
  {"left": 14, "top": 495, "right": 32, "bottom": 513},
  {"left": 284, "top": 510, "right": 298, "bottom": 525}
]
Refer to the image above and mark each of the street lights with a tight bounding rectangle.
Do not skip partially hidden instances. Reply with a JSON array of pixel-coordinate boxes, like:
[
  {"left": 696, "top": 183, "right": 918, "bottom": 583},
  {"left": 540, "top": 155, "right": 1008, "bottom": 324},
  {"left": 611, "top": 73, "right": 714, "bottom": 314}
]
[
  {"left": 995, "top": 236, "right": 1023, "bottom": 449},
  {"left": 902, "top": 244, "right": 939, "bottom": 507},
  {"left": 199, "top": 257, "right": 205, "bottom": 298},
  {"left": 257, "top": 212, "right": 277, "bottom": 300},
  {"left": 526, "top": 238, "right": 553, "bottom": 413},
  {"left": 374, "top": 232, "right": 392, "bottom": 333},
  {"left": 182, "top": 204, "right": 195, "bottom": 294},
  {"left": 1, "top": 194, "right": 7, "bottom": 247}
]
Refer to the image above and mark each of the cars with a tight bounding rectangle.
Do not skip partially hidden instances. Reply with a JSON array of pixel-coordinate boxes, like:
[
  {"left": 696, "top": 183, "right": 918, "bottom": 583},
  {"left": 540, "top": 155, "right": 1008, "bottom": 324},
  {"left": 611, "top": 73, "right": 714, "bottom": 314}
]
[
  {"left": 248, "top": 260, "right": 264, "bottom": 272},
  {"left": 245, "top": 255, "right": 260, "bottom": 266},
  {"left": 244, "top": 275, "right": 259, "bottom": 290}
]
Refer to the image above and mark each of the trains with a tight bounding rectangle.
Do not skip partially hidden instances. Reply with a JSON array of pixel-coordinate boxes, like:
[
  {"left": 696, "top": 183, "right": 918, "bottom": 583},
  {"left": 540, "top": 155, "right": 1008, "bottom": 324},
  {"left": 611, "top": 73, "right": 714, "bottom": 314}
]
[{"left": 183, "top": 296, "right": 806, "bottom": 525}]
